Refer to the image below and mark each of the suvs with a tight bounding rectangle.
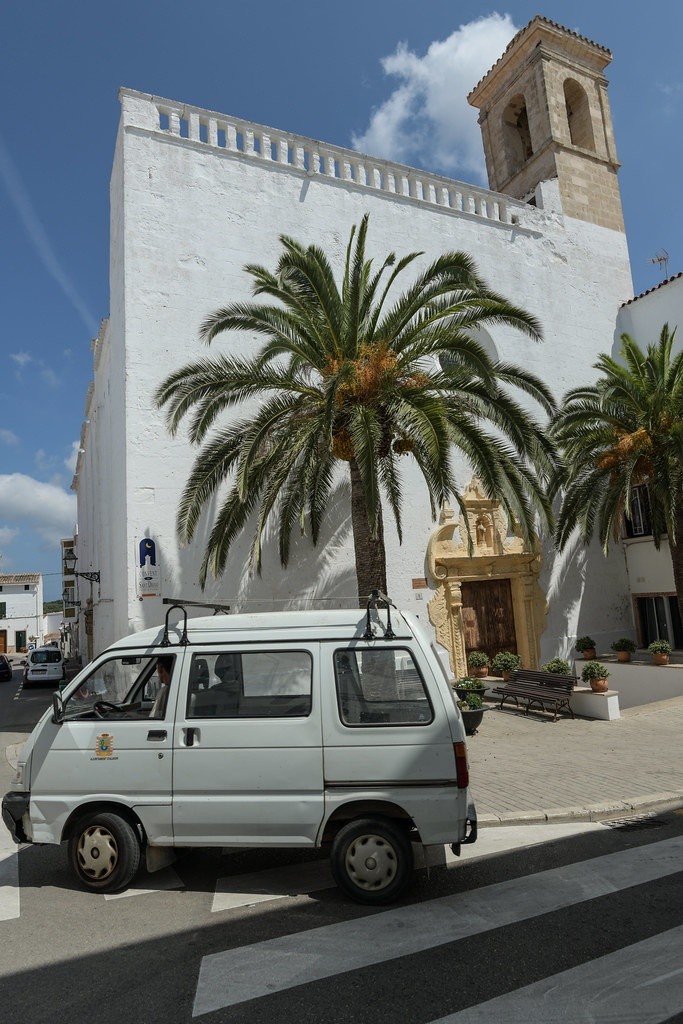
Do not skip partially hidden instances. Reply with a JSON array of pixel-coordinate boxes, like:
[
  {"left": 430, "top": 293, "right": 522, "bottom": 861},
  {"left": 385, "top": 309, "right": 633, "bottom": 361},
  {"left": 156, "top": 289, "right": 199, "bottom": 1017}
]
[{"left": 20, "top": 647, "right": 66, "bottom": 689}]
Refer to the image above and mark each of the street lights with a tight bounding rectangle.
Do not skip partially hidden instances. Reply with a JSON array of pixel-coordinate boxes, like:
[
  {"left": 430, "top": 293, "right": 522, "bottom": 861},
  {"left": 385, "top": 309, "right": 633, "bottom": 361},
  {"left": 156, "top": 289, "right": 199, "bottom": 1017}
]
[{"left": 61, "top": 589, "right": 69, "bottom": 658}]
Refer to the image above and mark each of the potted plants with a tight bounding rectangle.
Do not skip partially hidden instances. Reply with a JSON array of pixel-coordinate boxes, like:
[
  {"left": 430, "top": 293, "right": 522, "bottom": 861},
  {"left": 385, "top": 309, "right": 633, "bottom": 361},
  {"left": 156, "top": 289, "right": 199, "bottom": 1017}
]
[
  {"left": 610, "top": 638, "right": 637, "bottom": 662},
  {"left": 451, "top": 677, "right": 491, "bottom": 701},
  {"left": 574, "top": 636, "right": 596, "bottom": 659},
  {"left": 580, "top": 663, "right": 613, "bottom": 692},
  {"left": 492, "top": 651, "right": 521, "bottom": 681},
  {"left": 648, "top": 640, "right": 672, "bottom": 665},
  {"left": 455, "top": 692, "right": 491, "bottom": 734},
  {"left": 468, "top": 651, "right": 491, "bottom": 677}
]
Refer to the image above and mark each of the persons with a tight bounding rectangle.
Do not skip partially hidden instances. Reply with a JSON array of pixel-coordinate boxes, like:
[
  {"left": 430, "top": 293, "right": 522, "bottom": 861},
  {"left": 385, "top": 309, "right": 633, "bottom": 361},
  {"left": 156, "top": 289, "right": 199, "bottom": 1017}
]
[{"left": 122, "top": 655, "right": 175, "bottom": 719}]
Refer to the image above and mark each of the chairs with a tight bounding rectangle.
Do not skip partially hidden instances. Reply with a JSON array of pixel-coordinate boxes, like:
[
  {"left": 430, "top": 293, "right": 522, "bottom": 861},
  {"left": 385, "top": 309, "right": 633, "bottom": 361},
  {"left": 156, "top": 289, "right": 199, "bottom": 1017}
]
[
  {"left": 190, "top": 660, "right": 218, "bottom": 717},
  {"left": 211, "top": 654, "right": 242, "bottom": 716}
]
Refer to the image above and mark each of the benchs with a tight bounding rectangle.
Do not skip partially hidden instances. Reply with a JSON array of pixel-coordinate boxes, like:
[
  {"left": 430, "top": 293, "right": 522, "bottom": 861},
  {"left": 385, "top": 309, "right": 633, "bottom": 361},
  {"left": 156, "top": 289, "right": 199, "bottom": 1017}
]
[{"left": 492, "top": 669, "right": 580, "bottom": 722}]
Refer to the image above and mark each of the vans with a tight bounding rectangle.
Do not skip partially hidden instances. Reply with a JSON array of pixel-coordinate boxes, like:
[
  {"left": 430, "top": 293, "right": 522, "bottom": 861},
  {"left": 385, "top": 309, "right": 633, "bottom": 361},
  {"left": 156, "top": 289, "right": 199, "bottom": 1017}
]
[{"left": 1, "top": 589, "right": 477, "bottom": 904}]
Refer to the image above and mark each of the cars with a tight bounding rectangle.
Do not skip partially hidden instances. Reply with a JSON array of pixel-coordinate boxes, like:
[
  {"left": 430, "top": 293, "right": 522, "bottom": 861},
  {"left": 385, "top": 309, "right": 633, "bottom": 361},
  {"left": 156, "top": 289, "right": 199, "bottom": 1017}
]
[{"left": 0, "top": 655, "right": 13, "bottom": 681}]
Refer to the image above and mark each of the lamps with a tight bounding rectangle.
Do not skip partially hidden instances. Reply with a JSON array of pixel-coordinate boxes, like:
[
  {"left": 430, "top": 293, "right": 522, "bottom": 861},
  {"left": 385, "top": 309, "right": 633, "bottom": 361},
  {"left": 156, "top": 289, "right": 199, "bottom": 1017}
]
[
  {"left": 61, "top": 589, "right": 82, "bottom": 606},
  {"left": 62, "top": 549, "right": 100, "bottom": 584}
]
